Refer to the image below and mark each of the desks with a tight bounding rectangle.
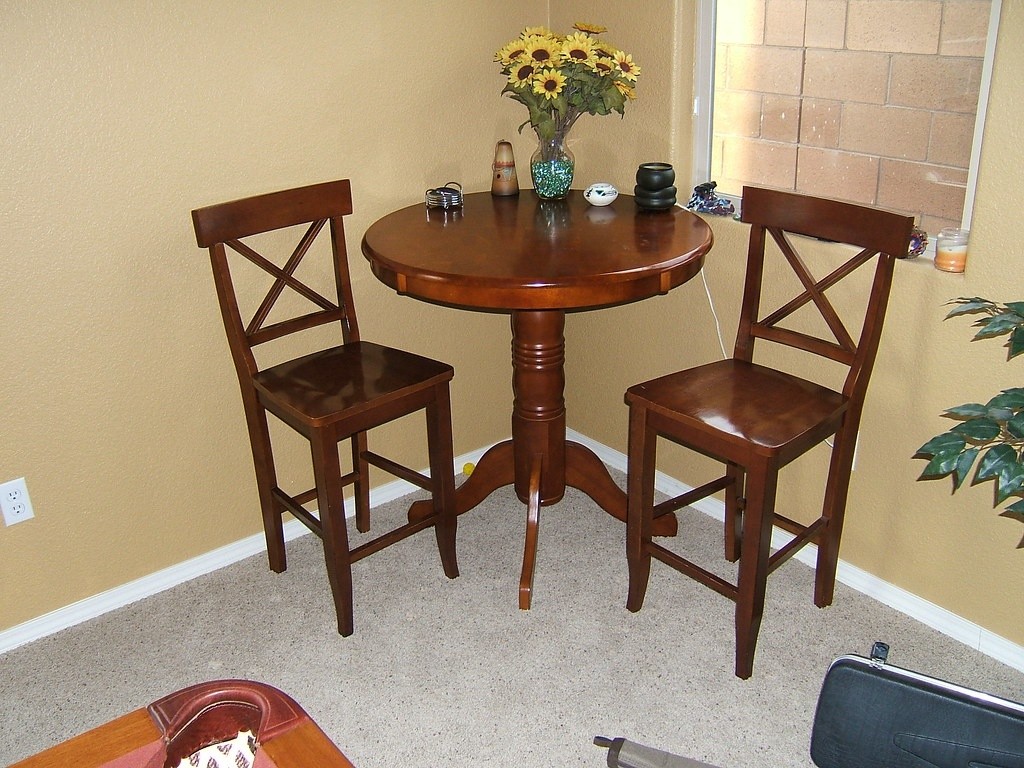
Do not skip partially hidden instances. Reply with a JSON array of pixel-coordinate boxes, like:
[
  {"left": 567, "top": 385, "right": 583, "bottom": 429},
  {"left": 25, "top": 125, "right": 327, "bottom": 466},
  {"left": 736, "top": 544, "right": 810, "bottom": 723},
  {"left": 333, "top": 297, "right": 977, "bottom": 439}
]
[{"left": 362, "top": 187, "right": 714, "bottom": 612}]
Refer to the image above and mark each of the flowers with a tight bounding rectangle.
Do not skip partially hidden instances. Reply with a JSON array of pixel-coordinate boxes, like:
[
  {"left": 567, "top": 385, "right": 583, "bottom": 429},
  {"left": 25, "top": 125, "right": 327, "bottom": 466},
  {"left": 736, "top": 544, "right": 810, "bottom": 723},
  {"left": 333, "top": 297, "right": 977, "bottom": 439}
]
[{"left": 494, "top": 18, "right": 642, "bottom": 161}]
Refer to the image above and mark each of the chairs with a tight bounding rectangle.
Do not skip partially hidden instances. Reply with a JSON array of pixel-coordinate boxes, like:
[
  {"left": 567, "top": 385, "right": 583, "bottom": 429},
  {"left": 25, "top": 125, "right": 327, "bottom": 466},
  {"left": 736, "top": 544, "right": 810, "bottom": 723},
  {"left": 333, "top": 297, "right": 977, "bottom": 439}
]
[
  {"left": 623, "top": 183, "right": 913, "bottom": 681},
  {"left": 192, "top": 178, "right": 462, "bottom": 639}
]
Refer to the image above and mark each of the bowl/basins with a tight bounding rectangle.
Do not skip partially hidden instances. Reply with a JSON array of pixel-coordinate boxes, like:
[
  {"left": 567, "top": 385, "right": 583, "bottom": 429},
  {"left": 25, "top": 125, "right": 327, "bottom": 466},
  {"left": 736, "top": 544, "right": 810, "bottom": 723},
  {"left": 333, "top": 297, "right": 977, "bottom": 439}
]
[{"left": 584, "top": 183, "right": 618, "bottom": 207}]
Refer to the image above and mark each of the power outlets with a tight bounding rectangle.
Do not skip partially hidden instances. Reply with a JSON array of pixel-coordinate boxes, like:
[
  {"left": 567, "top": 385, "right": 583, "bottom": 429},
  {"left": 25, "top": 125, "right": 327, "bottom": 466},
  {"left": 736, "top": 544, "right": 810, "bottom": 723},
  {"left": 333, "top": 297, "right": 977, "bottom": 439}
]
[{"left": 0, "top": 477, "right": 34, "bottom": 527}]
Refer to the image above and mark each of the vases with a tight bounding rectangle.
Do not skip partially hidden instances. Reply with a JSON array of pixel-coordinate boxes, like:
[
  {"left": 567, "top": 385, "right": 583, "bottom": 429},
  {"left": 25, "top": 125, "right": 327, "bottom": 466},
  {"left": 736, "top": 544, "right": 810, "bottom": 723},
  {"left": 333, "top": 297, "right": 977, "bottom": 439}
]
[{"left": 531, "top": 126, "right": 575, "bottom": 203}]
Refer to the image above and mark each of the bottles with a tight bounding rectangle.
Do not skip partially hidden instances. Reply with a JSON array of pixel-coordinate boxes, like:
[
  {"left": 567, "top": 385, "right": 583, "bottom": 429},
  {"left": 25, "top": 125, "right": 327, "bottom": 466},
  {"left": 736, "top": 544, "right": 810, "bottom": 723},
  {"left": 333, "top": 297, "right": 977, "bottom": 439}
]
[
  {"left": 633, "top": 162, "right": 677, "bottom": 214},
  {"left": 491, "top": 139, "right": 519, "bottom": 197},
  {"left": 934, "top": 227, "right": 969, "bottom": 273}
]
